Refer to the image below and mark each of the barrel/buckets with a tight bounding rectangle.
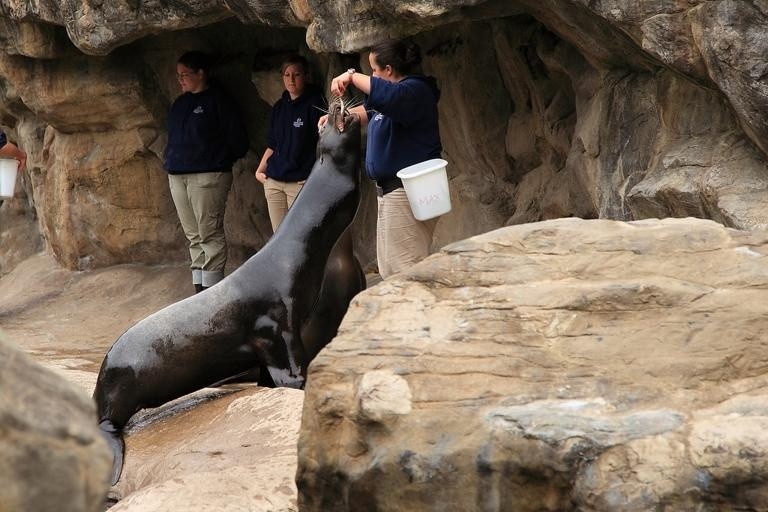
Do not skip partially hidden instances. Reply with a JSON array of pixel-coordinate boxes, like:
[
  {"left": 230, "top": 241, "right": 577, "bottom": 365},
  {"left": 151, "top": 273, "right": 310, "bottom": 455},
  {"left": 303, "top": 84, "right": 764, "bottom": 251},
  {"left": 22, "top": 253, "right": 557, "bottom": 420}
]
[{"left": 397, "top": 159, "right": 451, "bottom": 221}]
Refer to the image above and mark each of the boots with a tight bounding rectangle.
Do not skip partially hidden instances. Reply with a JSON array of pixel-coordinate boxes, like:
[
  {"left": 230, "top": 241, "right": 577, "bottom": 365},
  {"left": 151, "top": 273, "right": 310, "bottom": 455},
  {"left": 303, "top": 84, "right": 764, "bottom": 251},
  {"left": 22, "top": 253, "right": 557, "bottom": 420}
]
[{"left": 192, "top": 269, "right": 224, "bottom": 294}]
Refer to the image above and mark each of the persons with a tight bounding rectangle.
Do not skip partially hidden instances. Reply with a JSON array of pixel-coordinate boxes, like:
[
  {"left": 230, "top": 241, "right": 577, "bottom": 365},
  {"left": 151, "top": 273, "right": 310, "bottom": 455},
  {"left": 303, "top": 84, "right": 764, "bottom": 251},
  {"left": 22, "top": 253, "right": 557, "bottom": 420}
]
[
  {"left": 253, "top": 52, "right": 326, "bottom": 242},
  {"left": 314, "top": 39, "right": 449, "bottom": 282},
  {"left": 158, "top": 47, "right": 250, "bottom": 296}
]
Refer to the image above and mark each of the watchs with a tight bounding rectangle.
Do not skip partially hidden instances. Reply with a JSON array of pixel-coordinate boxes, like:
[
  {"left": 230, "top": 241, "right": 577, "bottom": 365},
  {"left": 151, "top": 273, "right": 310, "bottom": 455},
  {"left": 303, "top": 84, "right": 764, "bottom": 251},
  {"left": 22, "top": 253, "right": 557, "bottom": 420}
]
[{"left": 346, "top": 68, "right": 356, "bottom": 84}]
